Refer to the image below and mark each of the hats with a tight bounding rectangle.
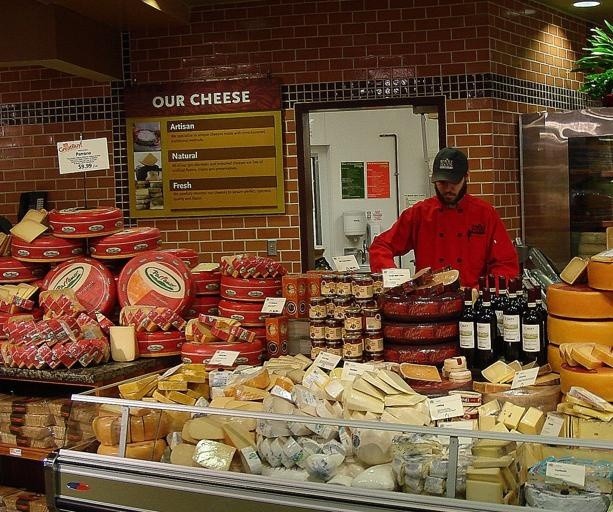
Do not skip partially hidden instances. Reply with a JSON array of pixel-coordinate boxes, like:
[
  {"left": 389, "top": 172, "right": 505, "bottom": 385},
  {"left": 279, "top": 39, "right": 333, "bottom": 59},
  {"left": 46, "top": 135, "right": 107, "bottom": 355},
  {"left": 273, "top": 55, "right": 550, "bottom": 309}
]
[{"left": 431, "top": 147, "right": 468, "bottom": 183}]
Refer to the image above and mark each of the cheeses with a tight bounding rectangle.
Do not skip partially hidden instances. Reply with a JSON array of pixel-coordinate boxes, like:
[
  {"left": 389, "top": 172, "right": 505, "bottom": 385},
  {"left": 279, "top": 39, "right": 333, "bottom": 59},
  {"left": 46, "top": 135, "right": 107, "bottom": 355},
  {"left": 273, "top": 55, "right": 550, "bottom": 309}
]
[{"left": 0, "top": 253, "right": 613, "bottom": 512}]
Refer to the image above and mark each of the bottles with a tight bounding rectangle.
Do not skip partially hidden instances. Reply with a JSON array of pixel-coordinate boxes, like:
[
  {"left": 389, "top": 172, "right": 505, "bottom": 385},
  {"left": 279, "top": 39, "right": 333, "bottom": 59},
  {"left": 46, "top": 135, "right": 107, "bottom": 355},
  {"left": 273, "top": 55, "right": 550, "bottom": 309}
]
[{"left": 459, "top": 273, "right": 547, "bottom": 368}]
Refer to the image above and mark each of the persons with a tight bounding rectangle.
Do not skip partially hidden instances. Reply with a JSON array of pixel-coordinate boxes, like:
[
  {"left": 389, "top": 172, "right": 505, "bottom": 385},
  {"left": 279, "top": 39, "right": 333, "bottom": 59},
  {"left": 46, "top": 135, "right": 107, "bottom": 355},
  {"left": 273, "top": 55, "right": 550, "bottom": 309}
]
[{"left": 369, "top": 147, "right": 520, "bottom": 293}]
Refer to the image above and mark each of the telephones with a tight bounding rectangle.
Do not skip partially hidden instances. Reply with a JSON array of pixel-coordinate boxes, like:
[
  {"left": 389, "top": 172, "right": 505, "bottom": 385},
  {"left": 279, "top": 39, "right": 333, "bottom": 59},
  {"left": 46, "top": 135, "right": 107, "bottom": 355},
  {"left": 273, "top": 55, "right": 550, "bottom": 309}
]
[{"left": 19, "top": 192, "right": 47, "bottom": 225}]
